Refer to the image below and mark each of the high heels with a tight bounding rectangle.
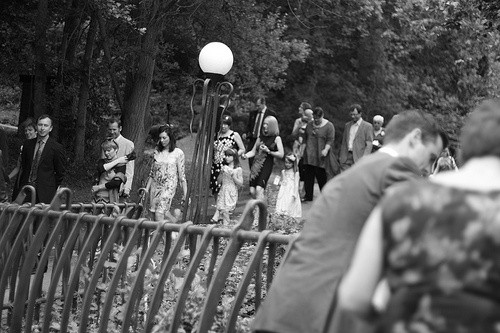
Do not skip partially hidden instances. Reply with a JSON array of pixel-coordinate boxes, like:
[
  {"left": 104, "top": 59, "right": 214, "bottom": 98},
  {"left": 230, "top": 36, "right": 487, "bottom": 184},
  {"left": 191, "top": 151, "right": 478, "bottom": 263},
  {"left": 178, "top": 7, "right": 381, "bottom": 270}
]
[{"left": 210, "top": 218, "right": 223, "bottom": 224}]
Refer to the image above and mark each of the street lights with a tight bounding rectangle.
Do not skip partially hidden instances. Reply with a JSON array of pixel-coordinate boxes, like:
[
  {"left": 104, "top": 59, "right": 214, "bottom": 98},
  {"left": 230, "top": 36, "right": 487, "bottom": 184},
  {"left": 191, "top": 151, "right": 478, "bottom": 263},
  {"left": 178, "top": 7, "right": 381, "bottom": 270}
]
[{"left": 188, "top": 40, "right": 236, "bottom": 219}]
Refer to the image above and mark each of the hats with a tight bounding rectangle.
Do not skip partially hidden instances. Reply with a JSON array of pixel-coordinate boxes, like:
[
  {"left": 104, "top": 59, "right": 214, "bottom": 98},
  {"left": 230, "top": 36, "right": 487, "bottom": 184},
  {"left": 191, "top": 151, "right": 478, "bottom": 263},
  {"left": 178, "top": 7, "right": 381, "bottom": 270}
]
[{"left": 350, "top": 113, "right": 361, "bottom": 117}]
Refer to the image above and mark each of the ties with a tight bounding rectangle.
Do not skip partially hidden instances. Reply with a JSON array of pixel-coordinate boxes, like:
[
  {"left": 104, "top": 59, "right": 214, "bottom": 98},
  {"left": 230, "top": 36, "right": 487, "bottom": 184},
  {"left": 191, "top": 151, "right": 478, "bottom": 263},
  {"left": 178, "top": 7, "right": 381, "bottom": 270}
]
[{"left": 31, "top": 141, "right": 45, "bottom": 182}]
